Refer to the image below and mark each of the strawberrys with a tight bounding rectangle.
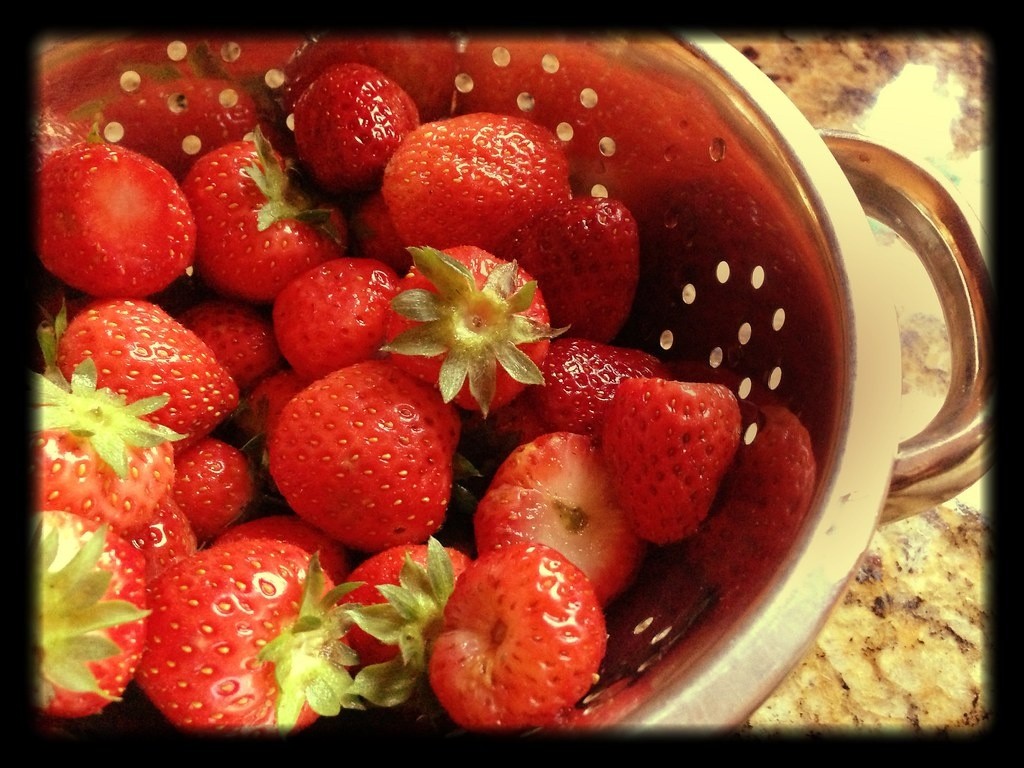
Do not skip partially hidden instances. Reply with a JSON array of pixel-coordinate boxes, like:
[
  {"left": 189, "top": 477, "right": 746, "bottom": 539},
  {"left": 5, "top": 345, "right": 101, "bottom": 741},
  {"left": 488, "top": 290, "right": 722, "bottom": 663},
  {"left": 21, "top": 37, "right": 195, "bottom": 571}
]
[{"left": 30, "top": 35, "right": 817, "bottom": 735}]
